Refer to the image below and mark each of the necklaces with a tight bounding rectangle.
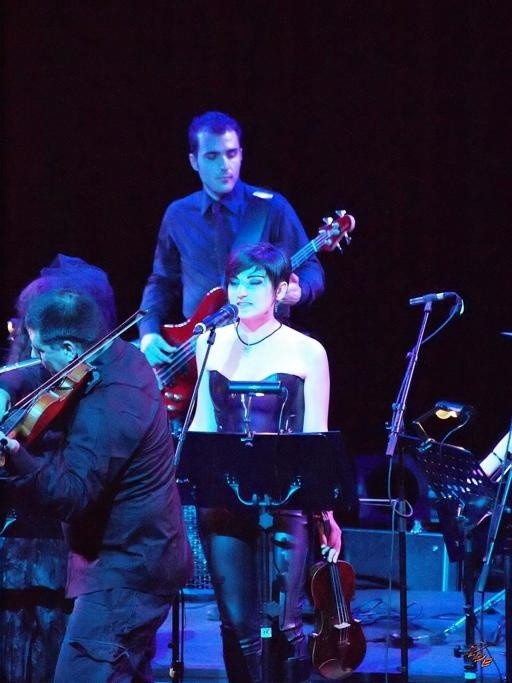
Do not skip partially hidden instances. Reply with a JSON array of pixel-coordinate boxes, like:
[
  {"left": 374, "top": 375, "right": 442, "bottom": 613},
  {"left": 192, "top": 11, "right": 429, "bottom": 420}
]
[{"left": 235, "top": 320, "right": 284, "bottom": 356}]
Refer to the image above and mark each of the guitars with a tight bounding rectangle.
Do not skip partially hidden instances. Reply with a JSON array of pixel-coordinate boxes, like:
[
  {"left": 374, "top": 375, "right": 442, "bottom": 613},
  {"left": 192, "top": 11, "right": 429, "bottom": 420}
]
[{"left": 149, "top": 209, "right": 357, "bottom": 420}]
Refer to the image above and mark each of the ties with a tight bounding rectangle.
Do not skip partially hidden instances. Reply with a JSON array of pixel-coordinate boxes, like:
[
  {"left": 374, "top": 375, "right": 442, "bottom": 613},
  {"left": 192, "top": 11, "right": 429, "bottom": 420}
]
[{"left": 212, "top": 203, "right": 229, "bottom": 290}]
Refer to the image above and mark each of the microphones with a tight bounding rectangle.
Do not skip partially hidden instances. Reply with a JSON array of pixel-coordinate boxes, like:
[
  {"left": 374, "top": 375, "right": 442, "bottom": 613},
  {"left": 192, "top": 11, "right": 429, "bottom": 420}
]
[{"left": 192, "top": 304, "right": 238, "bottom": 334}]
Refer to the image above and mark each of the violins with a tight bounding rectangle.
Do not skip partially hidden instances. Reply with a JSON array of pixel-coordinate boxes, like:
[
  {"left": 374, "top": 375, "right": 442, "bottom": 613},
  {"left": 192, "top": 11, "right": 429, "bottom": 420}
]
[
  {"left": 1, "top": 304, "right": 152, "bottom": 474},
  {"left": 310, "top": 512, "right": 365, "bottom": 681}
]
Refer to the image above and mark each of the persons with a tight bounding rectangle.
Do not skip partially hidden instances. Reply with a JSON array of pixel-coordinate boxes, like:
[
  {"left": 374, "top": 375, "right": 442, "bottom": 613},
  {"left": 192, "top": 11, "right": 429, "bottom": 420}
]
[
  {"left": 135, "top": 107, "right": 327, "bottom": 683},
  {"left": 185, "top": 241, "right": 343, "bottom": 682},
  {"left": 1, "top": 287, "right": 193, "bottom": 683}
]
